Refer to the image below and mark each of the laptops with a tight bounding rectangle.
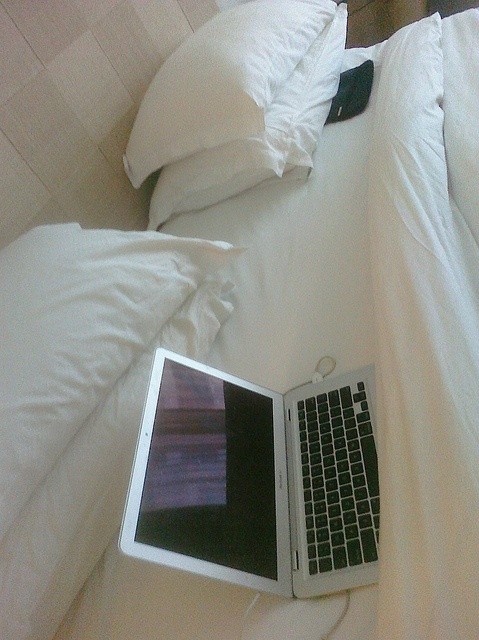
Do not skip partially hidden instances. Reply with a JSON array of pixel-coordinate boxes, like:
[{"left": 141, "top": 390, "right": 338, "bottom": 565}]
[{"left": 117, "top": 345, "right": 381, "bottom": 601}]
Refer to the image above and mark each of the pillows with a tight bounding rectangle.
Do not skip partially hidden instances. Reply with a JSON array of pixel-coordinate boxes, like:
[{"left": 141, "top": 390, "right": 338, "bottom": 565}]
[
  {"left": 122, "top": 1, "right": 340, "bottom": 190},
  {"left": 146, "top": 2, "right": 349, "bottom": 232},
  {"left": 1, "top": 221, "right": 246, "bottom": 541},
  {"left": 1, "top": 277, "right": 234, "bottom": 639}
]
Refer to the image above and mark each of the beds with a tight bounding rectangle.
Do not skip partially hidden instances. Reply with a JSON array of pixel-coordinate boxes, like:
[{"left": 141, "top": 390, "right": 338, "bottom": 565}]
[{"left": 53, "top": 7, "right": 478, "bottom": 638}]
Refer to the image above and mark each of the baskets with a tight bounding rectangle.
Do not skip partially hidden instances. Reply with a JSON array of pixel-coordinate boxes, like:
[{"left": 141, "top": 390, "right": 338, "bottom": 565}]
[{"left": 325, "top": 60, "right": 374, "bottom": 123}]
